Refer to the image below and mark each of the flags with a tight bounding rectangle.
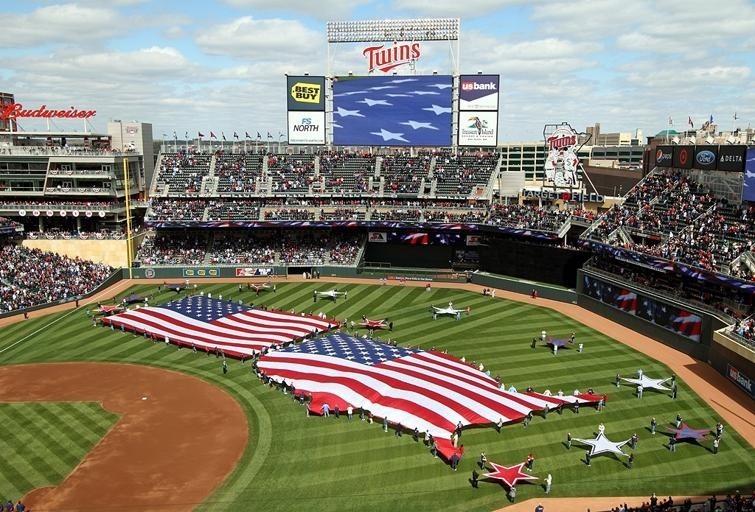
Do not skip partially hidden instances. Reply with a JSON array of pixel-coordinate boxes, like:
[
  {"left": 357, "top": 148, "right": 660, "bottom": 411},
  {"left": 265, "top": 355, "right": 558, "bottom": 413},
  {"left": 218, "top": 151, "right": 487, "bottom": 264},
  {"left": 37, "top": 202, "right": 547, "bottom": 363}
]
[
  {"left": 710, "top": 114, "right": 714, "bottom": 124},
  {"left": 669, "top": 116, "right": 674, "bottom": 124},
  {"left": 162, "top": 130, "right": 286, "bottom": 139},
  {"left": 688, "top": 116, "right": 694, "bottom": 128}
]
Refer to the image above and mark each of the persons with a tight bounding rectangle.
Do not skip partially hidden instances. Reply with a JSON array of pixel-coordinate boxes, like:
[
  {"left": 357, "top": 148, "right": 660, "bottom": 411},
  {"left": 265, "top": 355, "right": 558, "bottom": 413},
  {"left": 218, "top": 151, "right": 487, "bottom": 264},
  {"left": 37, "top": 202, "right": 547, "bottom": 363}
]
[
  {"left": 0, "top": 500, "right": 3, "bottom": 511},
  {"left": 6, "top": 499, "right": 13, "bottom": 512},
  {"left": 15, "top": 500, "right": 24, "bottom": 512},
  {"left": 580, "top": 170, "right": 755, "bottom": 346},
  {"left": 369, "top": 299, "right": 471, "bottom": 356},
  {"left": 615, "top": 366, "right": 724, "bottom": 466},
  {"left": 531, "top": 327, "right": 585, "bottom": 357},
  {"left": 535, "top": 502, "right": 545, "bottom": 512},
  {"left": 91, "top": 317, "right": 369, "bottom": 356},
  {"left": 369, "top": 143, "right": 580, "bottom": 299},
  {"left": 611, "top": 488, "right": 755, "bottom": 512}
]
